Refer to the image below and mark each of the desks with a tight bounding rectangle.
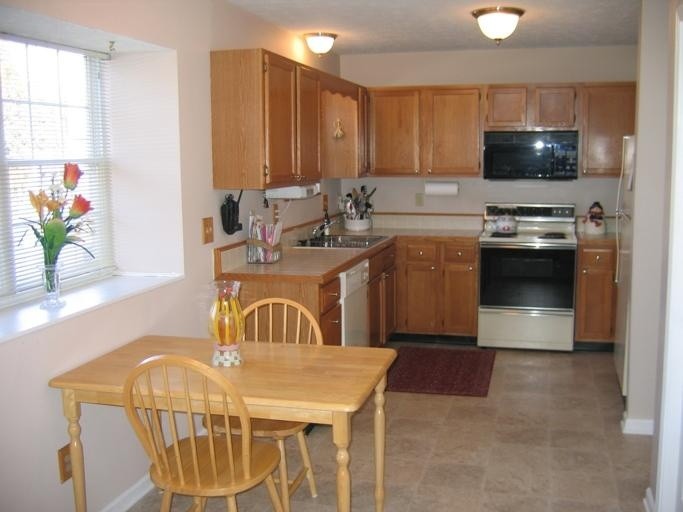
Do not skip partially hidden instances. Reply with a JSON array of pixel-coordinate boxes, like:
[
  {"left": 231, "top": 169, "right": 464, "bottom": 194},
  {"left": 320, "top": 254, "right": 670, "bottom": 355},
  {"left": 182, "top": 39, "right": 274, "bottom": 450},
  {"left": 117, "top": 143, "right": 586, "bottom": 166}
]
[{"left": 48, "top": 335, "right": 399, "bottom": 511}]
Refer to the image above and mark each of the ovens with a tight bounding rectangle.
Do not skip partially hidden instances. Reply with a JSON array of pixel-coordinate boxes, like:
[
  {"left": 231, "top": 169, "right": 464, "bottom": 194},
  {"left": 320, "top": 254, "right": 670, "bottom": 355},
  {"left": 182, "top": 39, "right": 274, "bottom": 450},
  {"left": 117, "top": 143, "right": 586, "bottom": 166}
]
[{"left": 477, "top": 240, "right": 576, "bottom": 354}]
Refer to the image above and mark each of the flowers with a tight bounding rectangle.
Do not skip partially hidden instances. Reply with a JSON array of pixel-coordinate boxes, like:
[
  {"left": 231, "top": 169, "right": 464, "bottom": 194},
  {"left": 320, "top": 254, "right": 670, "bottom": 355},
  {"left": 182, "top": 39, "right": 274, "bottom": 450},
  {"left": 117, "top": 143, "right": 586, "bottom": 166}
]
[{"left": 17, "top": 163, "right": 95, "bottom": 266}]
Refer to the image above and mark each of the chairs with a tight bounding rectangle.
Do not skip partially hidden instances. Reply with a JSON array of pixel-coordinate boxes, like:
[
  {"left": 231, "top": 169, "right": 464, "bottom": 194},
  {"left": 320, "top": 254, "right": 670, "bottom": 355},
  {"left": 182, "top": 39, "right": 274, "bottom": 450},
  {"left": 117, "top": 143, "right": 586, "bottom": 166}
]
[
  {"left": 201, "top": 298, "right": 324, "bottom": 511},
  {"left": 123, "top": 355, "right": 283, "bottom": 511}
]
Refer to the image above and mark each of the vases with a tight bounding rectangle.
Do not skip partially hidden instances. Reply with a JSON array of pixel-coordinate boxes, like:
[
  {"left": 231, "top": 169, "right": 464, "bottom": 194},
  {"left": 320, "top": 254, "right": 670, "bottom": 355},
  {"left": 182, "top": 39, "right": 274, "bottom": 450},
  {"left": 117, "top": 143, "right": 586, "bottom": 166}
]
[
  {"left": 38, "top": 262, "right": 63, "bottom": 308},
  {"left": 211, "top": 279, "right": 245, "bottom": 367}
]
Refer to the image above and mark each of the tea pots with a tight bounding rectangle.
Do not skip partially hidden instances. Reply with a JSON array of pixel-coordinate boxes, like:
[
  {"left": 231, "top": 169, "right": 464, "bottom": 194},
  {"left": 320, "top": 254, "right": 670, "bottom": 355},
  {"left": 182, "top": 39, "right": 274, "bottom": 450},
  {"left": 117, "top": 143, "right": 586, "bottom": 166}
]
[{"left": 488, "top": 205, "right": 520, "bottom": 235}]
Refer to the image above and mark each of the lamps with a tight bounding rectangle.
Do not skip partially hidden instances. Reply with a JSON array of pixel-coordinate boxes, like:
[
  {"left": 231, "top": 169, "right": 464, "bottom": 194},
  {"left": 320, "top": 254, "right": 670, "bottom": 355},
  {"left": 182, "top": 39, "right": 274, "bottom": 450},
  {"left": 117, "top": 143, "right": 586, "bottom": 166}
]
[
  {"left": 304, "top": 32, "right": 337, "bottom": 58},
  {"left": 470, "top": 7, "right": 525, "bottom": 46}
]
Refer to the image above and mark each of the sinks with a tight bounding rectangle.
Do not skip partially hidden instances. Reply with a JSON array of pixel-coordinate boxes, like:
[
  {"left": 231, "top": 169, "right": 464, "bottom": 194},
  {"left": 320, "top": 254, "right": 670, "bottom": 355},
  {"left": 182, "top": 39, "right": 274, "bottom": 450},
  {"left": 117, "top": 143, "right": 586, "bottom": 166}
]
[{"left": 287, "top": 228, "right": 390, "bottom": 251}]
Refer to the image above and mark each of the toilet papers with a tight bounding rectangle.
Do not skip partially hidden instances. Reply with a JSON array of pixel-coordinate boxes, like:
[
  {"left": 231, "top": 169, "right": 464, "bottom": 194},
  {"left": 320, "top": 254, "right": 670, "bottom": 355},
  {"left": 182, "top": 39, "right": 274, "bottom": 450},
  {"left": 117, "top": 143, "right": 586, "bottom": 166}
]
[{"left": 421, "top": 177, "right": 465, "bottom": 201}]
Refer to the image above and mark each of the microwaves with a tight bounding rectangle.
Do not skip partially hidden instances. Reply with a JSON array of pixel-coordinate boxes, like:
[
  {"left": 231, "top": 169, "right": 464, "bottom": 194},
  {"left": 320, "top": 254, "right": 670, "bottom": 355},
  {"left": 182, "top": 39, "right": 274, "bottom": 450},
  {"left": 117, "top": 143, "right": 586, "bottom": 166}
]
[{"left": 482, "top": 130, "right": 578, "bottom": 182}]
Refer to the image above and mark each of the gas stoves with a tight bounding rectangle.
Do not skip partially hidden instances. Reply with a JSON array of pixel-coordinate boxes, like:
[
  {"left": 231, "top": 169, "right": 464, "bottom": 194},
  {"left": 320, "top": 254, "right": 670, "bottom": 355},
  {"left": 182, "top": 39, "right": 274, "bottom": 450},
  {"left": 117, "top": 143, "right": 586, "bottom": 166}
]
[{"left": 479, "top": 228, "right": 576, "bottom": 243}]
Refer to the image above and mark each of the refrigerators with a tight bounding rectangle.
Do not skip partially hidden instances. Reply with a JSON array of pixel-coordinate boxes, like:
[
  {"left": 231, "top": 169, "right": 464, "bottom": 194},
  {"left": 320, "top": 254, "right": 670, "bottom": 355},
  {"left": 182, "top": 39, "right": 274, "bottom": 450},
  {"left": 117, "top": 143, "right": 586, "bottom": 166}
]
[{"left": 609, "top": 134, "right": 637, "bottom": 400}]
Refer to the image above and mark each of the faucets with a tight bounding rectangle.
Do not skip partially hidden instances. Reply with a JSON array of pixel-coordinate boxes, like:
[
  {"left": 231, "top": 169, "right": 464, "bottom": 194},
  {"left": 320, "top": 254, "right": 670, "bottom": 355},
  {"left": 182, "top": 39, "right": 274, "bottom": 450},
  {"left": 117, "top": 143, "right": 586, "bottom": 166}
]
[{"left": 309, "top": 215, "right": 343, "bottom": 241}]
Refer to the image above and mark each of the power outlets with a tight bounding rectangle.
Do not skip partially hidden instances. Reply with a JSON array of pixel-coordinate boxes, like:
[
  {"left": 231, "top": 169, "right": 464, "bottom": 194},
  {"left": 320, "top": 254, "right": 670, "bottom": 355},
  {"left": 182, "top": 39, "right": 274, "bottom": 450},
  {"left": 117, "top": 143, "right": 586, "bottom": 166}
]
[{"left": 272, "top": 203, "right": 279, "bottom": 225}]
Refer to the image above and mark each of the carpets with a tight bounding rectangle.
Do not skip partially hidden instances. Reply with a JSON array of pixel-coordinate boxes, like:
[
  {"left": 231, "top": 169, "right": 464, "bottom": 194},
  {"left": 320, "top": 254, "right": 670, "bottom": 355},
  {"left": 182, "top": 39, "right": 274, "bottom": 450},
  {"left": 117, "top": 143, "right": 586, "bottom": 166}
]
[{"left": 384, "top": 346, "right": 496, "bottom": 397}]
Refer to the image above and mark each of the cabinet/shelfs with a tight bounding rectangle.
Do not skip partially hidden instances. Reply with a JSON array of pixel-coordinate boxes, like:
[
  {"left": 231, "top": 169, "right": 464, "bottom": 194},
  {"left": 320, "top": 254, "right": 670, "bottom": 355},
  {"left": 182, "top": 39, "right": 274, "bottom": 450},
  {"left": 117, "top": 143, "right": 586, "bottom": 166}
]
[
  {"left": 366, "top": 85, "right": 482, "bottom": 177},
  {"left": 220, "top": 277, "right": 342, "bottom": 348},
  {"left": 440, "top": 238, "right": 478, "bottom": 336},
  {"left": 580, "top": 81, "right": 636, "bottom": 178},
  {"left": 211, "top": 47, "right": 320, "bottom": 190},
  {"left": 486, "top": 85, "right": 578, "bottom": 130},
  {"left": 368, "top": 243, "right": 396, "bottom": 348},
  {"left": 397, "top": 235, "right": 442, "bottom": 335},
  {"left": 320, "top": 73, "right": 369, "bottom": 179},
  {"left": 575, "top": 243, "right": 611, "bottom": 344}
]
